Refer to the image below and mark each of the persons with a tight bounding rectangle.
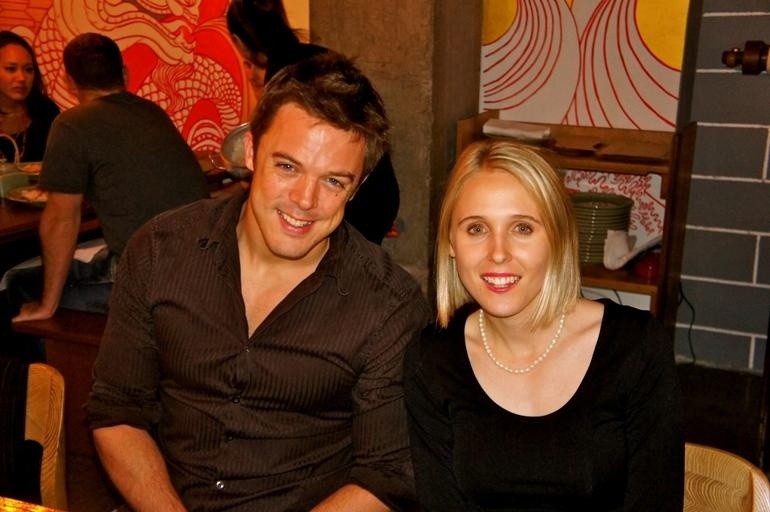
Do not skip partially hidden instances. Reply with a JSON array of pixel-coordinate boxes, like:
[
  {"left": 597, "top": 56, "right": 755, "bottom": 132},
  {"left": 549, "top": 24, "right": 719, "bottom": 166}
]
[
  {"left": 0, "top": 28, "right": 217, "bottom": 328},
  {"left": 225, "top": 0, "right": 403, "bottom": 253},
  {"left": 0, "top": 29, "right": 63, "bottom": 165},
  {"left": 399, "top": 136, "right": 687, "bottom": 512},
  {"left": 79, "top": 52, "right": 439, "bottom": 512}
]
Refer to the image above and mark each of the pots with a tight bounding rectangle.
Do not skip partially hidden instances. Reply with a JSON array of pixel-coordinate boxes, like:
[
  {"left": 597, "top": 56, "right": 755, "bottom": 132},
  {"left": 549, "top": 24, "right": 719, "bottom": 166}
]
[{"left": 209, "top": 122, "right": 256, "bottom": 178}]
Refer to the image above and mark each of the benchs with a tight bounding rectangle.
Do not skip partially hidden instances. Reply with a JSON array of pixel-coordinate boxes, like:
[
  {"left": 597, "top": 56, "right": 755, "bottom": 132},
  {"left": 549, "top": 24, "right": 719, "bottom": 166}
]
[{"left": 11, "top": 307, "right": 108, "bottom": 456}]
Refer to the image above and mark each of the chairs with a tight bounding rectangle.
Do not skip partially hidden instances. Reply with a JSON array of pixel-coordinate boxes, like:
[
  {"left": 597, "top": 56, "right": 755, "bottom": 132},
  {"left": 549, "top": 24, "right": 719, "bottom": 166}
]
[
  {"left": 25, "top": 363, "right": 67, "bottom": 511},
  {"left": 684, "top": 442, "right": 769, "bottom": 512}
]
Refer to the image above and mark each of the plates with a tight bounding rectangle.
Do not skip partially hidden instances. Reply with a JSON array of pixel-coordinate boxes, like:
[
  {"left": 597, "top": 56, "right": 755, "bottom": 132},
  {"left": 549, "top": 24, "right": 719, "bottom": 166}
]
[
  {"left": 4, "top": 185, "right": 47, "bottom": 209},
  {"left": 571, "top": 192, "right": 635, "bottom": 266},
  {"left": 16, "top": 160, "right": 42, "bottom": 181}
]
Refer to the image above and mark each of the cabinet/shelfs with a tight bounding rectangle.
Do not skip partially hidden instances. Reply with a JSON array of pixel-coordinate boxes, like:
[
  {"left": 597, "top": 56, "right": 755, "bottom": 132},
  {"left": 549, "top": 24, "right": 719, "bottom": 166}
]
[{"left": 455, "top": 107, "right": 698, "bottom": 327}]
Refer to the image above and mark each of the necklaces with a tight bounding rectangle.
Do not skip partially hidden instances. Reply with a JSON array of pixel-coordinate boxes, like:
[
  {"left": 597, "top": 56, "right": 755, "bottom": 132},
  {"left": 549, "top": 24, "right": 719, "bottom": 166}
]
[{"left": 478, "top": 307, "right": 569, "bottom": 376}]
